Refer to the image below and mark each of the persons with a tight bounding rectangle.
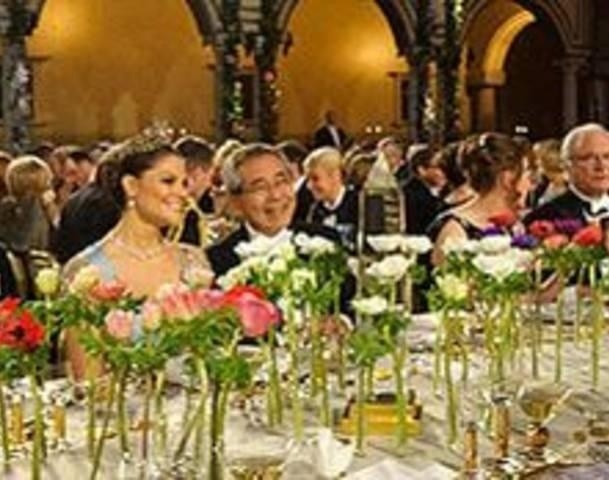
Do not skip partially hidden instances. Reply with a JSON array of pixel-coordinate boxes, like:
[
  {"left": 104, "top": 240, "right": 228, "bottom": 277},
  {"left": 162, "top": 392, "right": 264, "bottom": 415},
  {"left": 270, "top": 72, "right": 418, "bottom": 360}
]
[{"left": 1, "top": 109, "right": 608, "bottom": 377}]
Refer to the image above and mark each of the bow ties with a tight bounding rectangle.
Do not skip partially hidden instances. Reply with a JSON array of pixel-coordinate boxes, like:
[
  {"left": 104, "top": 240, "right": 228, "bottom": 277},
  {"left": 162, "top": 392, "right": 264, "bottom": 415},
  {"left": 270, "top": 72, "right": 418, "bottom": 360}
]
[
  {"left": 589, "top": 197, "right": 609, "bottom": 213},
  {"left": 320, "top": 204, "right": 344, "bottom": 218},
  {"left": 255, "top": 227, "right": 289, "bottom": 252}
]
